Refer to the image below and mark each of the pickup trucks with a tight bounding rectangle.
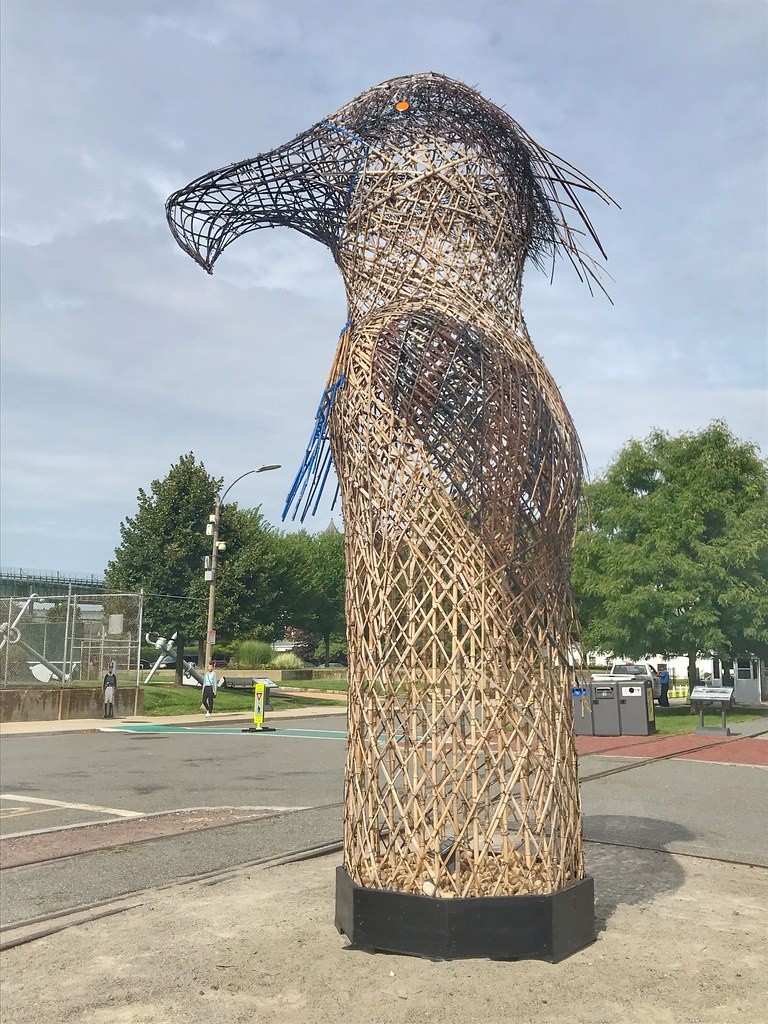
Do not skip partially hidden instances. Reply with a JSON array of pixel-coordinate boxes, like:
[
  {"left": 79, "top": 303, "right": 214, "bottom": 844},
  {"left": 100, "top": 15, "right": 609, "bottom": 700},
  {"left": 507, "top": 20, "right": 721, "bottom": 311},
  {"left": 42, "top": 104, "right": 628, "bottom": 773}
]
[{"left": 590, "top": 662, "right": 662, "bottom": 704}]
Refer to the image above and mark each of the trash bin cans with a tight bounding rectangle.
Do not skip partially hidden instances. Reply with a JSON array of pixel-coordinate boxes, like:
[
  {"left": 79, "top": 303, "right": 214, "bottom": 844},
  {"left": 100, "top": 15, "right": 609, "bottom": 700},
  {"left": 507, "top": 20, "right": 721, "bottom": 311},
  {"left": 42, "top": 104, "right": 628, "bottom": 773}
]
[
  {"left": 570, "top": 683, "right": 594, "bottom": 737},
  {"left": 590, "top": 682, "right": 621, "bottom": 737},
  {"left": 617, "top": 680, "right": 656, "bottom": 736}
]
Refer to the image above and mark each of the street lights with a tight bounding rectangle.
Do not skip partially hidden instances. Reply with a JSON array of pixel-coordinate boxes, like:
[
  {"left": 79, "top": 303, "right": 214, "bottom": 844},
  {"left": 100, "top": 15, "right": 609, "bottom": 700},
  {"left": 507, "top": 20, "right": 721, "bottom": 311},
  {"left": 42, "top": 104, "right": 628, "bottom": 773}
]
[{"left": 204, "top": 464, "right": 282, "bottom": 674}]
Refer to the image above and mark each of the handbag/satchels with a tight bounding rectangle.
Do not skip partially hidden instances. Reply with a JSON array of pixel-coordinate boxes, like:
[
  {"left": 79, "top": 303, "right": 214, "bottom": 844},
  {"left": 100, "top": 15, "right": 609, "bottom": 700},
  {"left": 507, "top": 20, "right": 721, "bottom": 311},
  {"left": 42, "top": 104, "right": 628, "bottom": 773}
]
[{"left": 213, "top": 693, "right": 216, "bottom": 698}]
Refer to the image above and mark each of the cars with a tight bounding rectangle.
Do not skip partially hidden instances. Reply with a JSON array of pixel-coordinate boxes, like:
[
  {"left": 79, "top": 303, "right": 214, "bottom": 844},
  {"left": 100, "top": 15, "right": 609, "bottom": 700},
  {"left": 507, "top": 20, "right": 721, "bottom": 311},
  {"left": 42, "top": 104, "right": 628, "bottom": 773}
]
[
  {"left": 318, "top": 663, "right": 345, "bottom": 668},
  {"left": 149, "top": 654, "right": 198, "bottom": 670}
]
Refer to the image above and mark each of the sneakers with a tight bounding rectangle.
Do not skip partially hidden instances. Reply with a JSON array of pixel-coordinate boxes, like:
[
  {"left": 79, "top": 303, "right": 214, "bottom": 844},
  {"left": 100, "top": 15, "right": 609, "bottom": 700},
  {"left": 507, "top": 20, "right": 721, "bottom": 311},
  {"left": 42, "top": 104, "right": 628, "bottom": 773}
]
[{"left": 206, "top": 710, "right": 212, "bottom": 718}]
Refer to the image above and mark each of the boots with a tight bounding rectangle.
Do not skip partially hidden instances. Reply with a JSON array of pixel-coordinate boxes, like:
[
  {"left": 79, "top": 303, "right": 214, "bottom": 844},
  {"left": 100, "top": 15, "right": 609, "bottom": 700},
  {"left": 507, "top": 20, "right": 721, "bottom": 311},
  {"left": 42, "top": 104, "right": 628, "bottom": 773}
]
[{"left": 103, "top": 703, "right": 112, "bottom": 718}]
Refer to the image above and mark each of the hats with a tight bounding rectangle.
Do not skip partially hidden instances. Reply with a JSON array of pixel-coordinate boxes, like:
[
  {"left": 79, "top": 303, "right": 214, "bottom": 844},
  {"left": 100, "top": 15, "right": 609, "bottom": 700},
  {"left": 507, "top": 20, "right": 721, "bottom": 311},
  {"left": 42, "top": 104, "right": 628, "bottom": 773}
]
[{"left": 109, "top": 662, "right": 113, "bottom": 668}]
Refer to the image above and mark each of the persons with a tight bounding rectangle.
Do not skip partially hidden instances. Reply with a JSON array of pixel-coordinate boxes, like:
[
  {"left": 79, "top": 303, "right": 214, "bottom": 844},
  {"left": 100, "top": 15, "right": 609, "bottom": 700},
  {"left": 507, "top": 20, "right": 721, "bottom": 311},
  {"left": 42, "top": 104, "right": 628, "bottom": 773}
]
[
  {"left": 656, "top": 665, "right": 669, "bottom": 707},
  {"left": 201, "top": 663, "right": 217, "bottom": 718},
  {"left": 102, "top": 662, "right": 116, "bottom": 719}
]
